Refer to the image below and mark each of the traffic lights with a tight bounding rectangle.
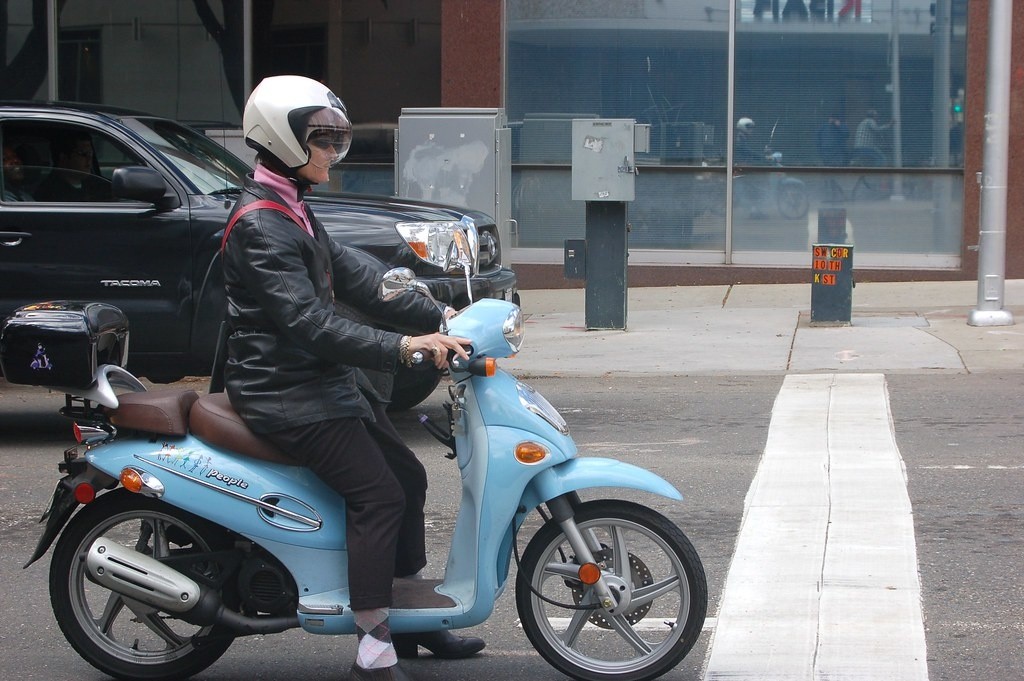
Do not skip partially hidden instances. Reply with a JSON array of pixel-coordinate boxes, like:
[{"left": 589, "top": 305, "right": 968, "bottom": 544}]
[{"left": 954, "top": 92, "right": 966, "bottom": 125}]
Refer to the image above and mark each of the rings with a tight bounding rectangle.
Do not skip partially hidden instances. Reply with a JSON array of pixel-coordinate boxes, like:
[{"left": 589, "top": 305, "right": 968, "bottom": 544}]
[{"left": 432, "top": 347, "right": 439, "bottom": 354}]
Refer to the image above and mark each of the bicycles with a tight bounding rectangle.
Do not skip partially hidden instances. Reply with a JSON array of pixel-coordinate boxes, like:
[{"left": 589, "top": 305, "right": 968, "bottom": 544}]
[{"left": 803, "top": 150, "right": 891, "bottom": 207}]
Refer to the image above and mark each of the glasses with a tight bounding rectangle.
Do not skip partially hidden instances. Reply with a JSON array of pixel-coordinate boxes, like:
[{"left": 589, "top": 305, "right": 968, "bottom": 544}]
[{"left": 309, "top": 132, "right": 344, "bottom": 154}]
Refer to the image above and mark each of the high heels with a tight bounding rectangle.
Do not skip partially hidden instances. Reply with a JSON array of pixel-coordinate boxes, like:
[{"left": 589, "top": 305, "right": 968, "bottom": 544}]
[
  {"left": 349, "top": 660, "right": 411, "bottom": 681},
  {"left": 391, "top": 622, "right": 485, "bottom": 659}
]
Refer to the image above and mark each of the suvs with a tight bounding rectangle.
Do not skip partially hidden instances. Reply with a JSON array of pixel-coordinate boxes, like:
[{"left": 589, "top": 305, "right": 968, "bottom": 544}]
[{"left": 0, "top": 96, "right": 525, "bottom": 412}]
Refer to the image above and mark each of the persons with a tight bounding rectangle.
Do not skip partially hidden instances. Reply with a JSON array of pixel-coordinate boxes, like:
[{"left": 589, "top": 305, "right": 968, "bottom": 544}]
[
  {"left": 44, "top": 128, "right": 96, "bottom": 202},
  {"left": 222, "top": 74, "right": 486, "bottom": 681},
  {"left": 855, "top": 110, "right": 895, "bottom": 167},
  {"left": 735, "top": 118, "right": 774, "bottom": 201},
  {"left": 817, "top": 111, "right": 850, "bottom": 168}
]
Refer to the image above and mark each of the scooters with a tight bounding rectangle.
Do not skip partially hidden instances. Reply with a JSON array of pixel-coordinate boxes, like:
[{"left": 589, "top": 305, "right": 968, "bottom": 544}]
[
  {"left": 693, "top": 145, "right": 812, "bottom": 225},
  {"left": 0, "top": 221, "right": 708, "bottom": 681}
]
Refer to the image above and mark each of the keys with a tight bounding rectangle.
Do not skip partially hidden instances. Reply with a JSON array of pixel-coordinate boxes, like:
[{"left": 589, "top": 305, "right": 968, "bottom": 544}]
[{"left": 452, "top": 409, "right": 462, "bottom": 422}]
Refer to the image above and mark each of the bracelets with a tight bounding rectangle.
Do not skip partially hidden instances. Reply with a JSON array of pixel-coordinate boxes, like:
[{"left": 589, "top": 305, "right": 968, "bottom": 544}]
[{"left": 400, "top": 334, "right": 412, "bottom": 367}]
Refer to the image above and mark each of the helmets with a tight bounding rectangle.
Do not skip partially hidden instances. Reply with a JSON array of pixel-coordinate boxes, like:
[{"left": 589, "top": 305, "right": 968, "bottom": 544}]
[
  {"left": 243, "top": 75, "right": 348, "bottom": 168},
  {"left": 736, "top": 117, "right": 755, "bottom": 134}
]
